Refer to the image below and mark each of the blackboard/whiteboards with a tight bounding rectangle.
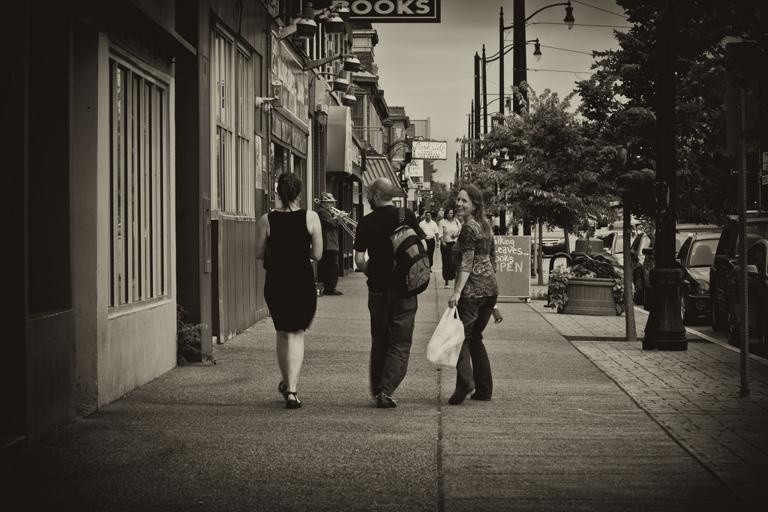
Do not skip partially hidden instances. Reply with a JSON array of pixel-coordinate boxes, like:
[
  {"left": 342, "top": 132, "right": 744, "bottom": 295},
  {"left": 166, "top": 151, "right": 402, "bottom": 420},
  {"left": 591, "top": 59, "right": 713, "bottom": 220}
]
[{"left": 494, "top": 236, "right": 531, "bottom": 297}]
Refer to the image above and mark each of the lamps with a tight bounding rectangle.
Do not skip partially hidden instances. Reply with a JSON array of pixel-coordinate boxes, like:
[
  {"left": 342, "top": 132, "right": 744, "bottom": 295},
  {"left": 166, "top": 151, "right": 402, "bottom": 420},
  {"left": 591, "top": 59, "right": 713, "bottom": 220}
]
[{"left": 272, "top": 0, "right": 360, "bottom": 106}]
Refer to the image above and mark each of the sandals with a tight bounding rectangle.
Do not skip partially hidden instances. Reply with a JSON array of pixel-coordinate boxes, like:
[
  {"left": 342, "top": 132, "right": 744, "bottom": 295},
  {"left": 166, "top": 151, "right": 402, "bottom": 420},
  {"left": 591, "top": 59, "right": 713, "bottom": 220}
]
[{"left": 278, "top": 380, "right": 303, "bottom": 409}]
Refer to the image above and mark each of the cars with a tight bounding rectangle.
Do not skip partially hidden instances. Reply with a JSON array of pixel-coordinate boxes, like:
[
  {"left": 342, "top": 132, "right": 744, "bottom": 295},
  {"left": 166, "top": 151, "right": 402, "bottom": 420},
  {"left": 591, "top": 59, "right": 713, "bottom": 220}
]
[{"left": 574, "top": 208, "right": 767, "bottom": 353}]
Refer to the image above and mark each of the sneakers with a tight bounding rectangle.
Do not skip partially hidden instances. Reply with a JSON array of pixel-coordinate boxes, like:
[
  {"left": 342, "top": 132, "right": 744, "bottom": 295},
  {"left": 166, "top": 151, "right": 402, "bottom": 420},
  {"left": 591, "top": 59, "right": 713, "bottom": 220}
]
[
  {"left": 322, "top": 289, "right": 343, "bottom": 295},
  {"left": 374, "top": 389, "right": 398, "bottom": 408},
  {"left": 449, "top": 382, "right": 491, "bottom": 404}
]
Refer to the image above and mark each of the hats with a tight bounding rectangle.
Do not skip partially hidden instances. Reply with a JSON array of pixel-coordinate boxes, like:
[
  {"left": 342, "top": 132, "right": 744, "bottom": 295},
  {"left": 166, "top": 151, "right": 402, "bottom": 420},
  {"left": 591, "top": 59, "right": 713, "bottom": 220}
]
[{"left": 320, "top": 193, "right": 338, "bottom": 202}]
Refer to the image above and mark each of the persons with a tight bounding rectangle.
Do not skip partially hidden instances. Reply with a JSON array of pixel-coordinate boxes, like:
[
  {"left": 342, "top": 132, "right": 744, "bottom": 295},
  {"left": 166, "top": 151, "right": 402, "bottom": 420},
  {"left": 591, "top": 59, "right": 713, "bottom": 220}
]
[
  {"left": 318, "top": 192, "right": 347, "bottom": 295},
  {"left": 446, "top": 183, "right": 498, "bottom": 405},
  {"left": 415, "top": 207, "right": 464, "bottom": 289},
  {"left": 253, "top": 172, "right": 323, "bottom": 409},
  {"left": 490, "top": 213, "right": 510, "bottom": 236},
  {"left": 353, "top": 176, "right": 428, "bottom": 407}
]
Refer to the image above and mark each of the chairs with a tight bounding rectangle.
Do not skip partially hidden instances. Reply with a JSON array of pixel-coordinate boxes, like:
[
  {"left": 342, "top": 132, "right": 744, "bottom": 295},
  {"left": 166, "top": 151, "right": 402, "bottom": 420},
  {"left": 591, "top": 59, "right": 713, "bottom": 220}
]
[{"left": 691, "top": 245, "right": 715, "bottom": 266}]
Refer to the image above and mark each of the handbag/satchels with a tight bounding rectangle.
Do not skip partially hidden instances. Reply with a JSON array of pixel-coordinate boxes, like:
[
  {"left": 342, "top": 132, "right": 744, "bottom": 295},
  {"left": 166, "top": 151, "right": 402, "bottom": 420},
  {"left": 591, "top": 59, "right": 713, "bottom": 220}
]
[{"left": 388, "top": 220, "right": 431, "bottom": 298}]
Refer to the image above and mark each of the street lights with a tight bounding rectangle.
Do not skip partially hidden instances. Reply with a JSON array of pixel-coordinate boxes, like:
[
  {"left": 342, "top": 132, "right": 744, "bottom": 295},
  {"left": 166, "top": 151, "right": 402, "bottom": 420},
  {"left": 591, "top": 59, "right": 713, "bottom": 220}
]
[{"left": 466, "top": 1, "right": 576, "bottom": 277}]
